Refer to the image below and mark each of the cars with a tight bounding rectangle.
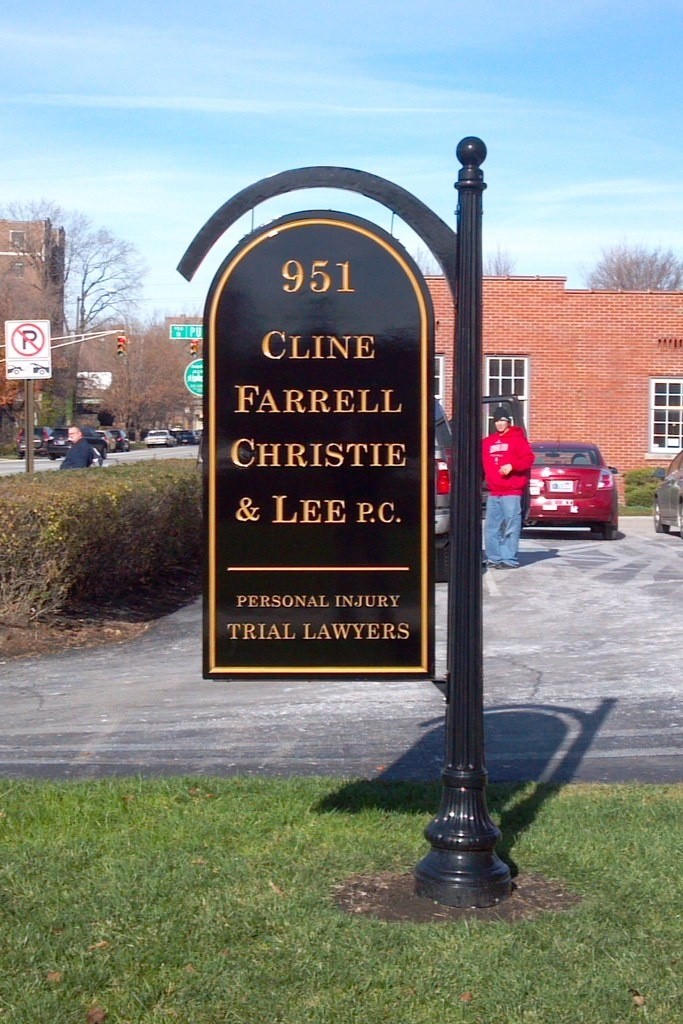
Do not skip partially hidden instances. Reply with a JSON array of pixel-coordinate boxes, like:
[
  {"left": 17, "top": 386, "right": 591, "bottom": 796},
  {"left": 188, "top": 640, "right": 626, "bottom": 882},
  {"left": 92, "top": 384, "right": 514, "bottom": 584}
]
[
  {"left": 435, "top": 393, "right": 683, "bottom": 580},
  {"left": 18, "top": 426, "right": 202, "bottom": 461}
]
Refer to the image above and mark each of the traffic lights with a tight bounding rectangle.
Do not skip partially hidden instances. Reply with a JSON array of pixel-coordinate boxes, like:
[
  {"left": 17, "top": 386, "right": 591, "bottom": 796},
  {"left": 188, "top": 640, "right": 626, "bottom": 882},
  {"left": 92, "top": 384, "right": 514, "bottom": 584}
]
[
  {"left": 117, "top": 336, "right": 126, "bottom": 358},
  {"left": 190, "top": 339, "right": 198, "bottom": 359}
]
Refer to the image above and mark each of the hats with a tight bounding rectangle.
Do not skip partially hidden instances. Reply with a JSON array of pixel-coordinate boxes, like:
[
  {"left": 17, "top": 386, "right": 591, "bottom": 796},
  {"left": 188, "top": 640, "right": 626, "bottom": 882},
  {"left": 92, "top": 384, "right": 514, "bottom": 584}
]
[{"left": 494, "top": 407, "right": 511, "bottom": 421}]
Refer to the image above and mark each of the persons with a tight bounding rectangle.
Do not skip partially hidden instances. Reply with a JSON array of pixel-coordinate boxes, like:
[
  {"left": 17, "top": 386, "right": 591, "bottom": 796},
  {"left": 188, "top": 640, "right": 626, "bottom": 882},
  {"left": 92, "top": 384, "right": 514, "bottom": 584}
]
[
  {"left": 481, "top": 407, "right": 536, "bottom": 569},
  {"left": 60, "top": 423, "right": 94, "bottom": 470}
]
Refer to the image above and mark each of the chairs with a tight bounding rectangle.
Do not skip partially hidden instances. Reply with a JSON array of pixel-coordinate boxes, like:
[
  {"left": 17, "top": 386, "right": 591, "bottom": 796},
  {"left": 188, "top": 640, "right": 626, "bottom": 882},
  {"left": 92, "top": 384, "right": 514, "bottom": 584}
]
[{"left": 573, "top": 457, "right": 588, "bottom": 464}]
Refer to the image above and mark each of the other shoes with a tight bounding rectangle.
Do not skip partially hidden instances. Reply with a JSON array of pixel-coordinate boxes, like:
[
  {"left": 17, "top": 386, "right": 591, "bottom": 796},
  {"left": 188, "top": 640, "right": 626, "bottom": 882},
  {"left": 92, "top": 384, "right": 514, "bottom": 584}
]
[
  {"left": 494, "top": 561, "right": 514, "bottom": 569},
  {"left": 482, "top": 559, "right": 496, "bottom": 568}
]
